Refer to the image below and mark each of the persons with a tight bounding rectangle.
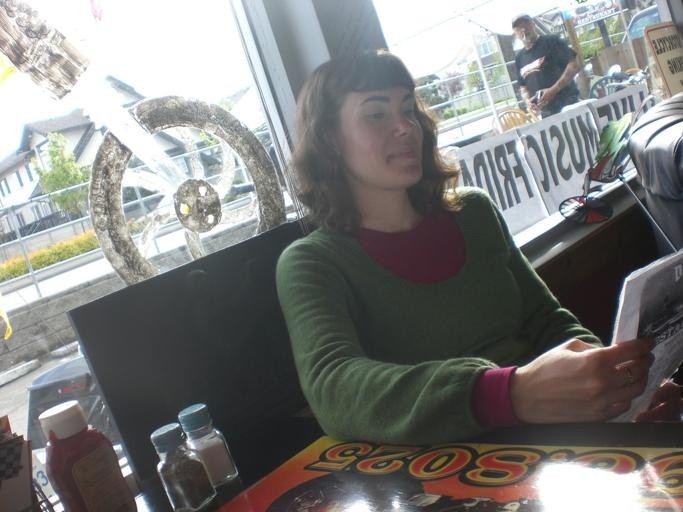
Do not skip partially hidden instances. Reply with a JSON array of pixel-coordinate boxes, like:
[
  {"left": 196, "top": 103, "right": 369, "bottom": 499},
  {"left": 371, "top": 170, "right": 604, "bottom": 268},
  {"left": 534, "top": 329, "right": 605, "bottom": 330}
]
[
  {"left": 275, "top": 45, "right": 676, "bottom": 446},
  {"left": 510, "top": 14, "right": 580, "bottom": 119}
]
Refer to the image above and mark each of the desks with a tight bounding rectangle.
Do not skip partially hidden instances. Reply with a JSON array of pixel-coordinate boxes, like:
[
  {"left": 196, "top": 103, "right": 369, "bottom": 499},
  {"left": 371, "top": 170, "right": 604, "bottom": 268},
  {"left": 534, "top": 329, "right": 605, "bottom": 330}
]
[{"left": 130, "top": 418, "right": 683, "bottom": 511}]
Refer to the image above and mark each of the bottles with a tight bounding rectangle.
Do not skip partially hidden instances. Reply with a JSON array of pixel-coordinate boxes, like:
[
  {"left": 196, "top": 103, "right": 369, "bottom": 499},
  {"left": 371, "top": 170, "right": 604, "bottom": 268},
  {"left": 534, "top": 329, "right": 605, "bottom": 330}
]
[
  {"left": 38, "top": 400, "right": 138, "bottom": 512},
  {"left": 177, "top": 403, "right": 239, "bottom": 488},
  {"left": 150, "top": 423, "right": 218, "bottom": 512}
]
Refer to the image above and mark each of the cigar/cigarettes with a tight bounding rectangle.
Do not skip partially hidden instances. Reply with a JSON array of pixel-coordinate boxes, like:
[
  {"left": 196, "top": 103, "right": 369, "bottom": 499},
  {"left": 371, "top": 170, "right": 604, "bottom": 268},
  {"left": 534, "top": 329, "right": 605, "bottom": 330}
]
[{"left": 523, "top": 33, "right": 527, "bottom": 41}]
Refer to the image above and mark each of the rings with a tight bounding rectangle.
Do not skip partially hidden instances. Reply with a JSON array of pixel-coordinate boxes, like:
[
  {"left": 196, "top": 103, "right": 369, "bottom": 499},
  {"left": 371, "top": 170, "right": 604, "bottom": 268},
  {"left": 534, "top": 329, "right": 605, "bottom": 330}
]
[{"left": 622, "top": 366, "right": 636, "bottom": 386}]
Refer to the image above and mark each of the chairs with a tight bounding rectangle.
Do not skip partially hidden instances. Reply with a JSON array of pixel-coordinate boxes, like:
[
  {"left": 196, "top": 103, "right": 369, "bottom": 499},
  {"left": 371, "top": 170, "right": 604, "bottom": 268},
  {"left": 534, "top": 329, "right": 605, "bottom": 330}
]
[{"left": 68, "top": 203, "right": 340, "bottom": 505}]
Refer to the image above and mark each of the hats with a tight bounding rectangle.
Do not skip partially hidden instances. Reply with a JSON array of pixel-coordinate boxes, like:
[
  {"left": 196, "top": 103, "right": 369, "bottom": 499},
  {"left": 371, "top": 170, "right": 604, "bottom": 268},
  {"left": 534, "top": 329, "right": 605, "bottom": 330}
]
[{"left": 512, "top": 14, "right": 533, "bottom": 28}]
